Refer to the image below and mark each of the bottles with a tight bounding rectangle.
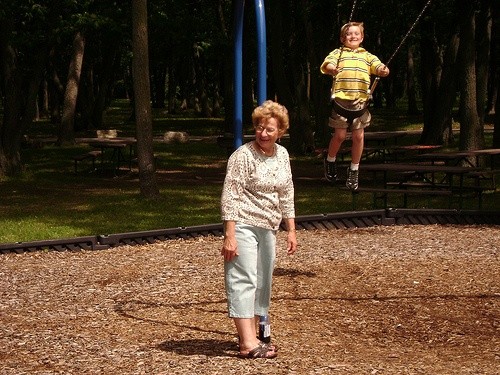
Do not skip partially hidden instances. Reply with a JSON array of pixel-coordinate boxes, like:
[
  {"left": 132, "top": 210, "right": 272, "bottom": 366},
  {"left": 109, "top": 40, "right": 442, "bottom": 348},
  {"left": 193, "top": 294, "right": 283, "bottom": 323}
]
[{"left": 259, "top": 303, "right": 271, "bottom": 344}]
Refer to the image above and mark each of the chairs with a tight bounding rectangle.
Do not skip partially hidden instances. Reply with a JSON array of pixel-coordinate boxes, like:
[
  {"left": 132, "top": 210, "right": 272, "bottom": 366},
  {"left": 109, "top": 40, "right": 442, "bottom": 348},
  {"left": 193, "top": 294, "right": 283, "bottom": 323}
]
[{"left": 74, "top": 129, "right": 127, "bottom": 172}]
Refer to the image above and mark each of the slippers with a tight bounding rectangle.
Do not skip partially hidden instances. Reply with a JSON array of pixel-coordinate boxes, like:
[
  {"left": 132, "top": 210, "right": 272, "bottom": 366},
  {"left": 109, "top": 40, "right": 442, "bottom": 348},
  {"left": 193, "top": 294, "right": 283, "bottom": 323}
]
[{"left": 238, "top": 341, "right": 279, "bottom": 358}]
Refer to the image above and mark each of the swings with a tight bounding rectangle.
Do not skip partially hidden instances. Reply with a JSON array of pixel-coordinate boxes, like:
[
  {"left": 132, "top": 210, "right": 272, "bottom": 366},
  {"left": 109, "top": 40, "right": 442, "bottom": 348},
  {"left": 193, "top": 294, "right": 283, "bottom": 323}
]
[{"left": 330, "top": 0, "right": 432, "bottom": 126}]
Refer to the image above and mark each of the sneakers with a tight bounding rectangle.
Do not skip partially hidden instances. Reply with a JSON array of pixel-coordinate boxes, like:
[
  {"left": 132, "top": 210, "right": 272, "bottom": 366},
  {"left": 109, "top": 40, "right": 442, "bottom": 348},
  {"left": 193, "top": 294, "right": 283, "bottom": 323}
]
[{"left": 324, "top": 157, "right": 337, "bottom": 182}]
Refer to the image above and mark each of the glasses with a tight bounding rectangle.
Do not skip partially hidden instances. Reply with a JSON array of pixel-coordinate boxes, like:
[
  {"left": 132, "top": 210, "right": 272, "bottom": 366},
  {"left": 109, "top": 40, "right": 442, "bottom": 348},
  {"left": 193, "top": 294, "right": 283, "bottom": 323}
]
[{"left": 346, "top": 167, "right": 359, "bottom": 191}]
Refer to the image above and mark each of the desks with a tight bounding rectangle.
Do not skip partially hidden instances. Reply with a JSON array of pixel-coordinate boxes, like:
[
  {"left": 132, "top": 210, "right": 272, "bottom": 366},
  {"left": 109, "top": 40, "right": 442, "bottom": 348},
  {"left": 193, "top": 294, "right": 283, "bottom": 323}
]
[
  {"left": 343, "top": 163, "right": 484, "bottom": 210},
  {"left": 331, "top": 130, "right": 407, "bottom": 164},
  {"left": 415, "top": 152, "right": 473, "bottom": 186},
  {"left": 473, "top": 149, "right": 500, "bottom": 189},
  {"left": 75, "top": 137, "right": 137, "bottom": 174}
]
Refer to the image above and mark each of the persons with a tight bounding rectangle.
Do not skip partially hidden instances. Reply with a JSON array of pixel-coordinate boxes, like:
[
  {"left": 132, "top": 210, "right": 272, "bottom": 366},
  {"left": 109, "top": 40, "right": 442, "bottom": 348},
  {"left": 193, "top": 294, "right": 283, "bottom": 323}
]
[
  {"left": 319, "top": 21, "right": 390, "bottom": 191},
  {"left": 219, "top": 99, "right": 298, "bottom": 359}
]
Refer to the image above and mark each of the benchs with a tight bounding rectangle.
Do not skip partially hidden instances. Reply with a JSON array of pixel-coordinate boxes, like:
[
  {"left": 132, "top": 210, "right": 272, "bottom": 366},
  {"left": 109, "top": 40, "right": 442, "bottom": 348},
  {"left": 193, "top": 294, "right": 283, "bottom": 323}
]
[
  {"left": 337, "top": 187, "right": 452, "bottom": 195},
  {"left": 387, "top": 181, "right": 466, "bottom": 193}
]
